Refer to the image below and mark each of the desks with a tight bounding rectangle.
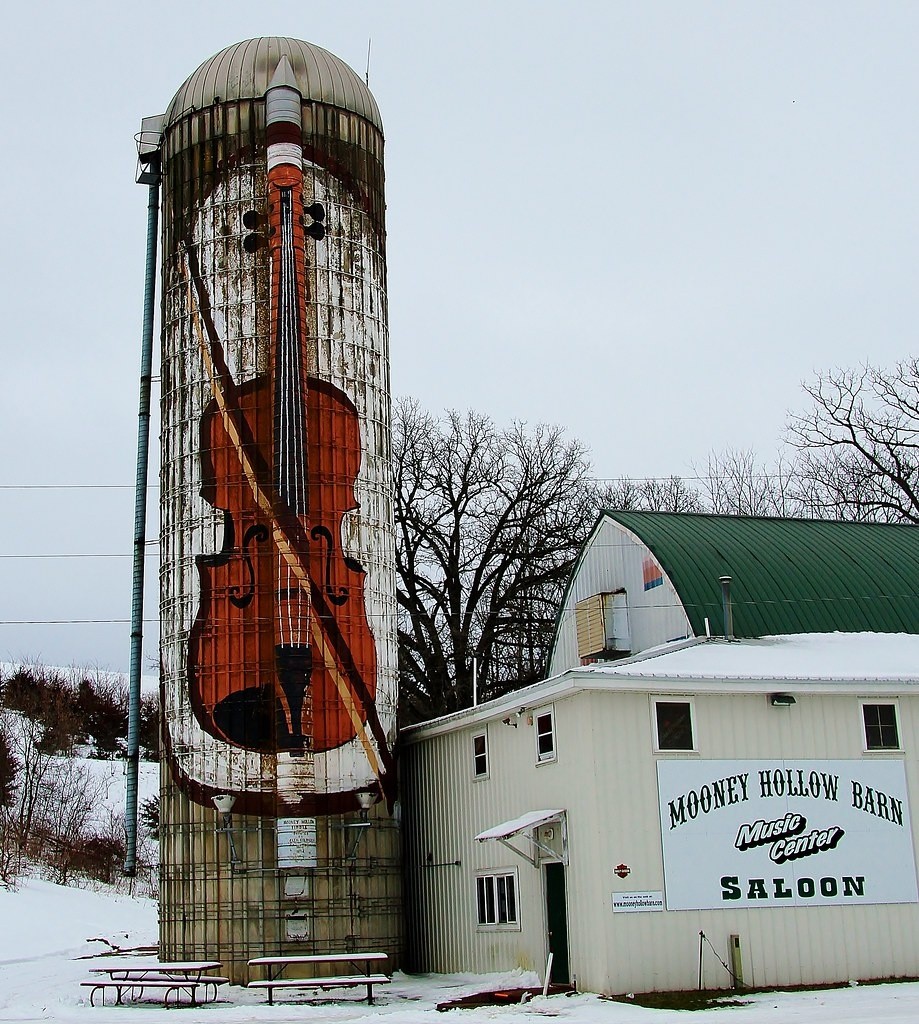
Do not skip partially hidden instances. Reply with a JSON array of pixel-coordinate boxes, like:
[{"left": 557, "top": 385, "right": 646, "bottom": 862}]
[
  {"left": 88, "top": 961, "right": 223, "bottom": 1007},
  {"left": 248, "top": 953, "right": 388, "bottom": 1006}
]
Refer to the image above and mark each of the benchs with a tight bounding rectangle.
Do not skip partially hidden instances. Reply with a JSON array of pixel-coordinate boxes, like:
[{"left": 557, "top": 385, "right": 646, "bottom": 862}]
[
  {"left": 80, "top": 974, "right": 231, "bottom": 987},
  {"left": 248, "top": 973, "right": 392, "bottom": 988}
]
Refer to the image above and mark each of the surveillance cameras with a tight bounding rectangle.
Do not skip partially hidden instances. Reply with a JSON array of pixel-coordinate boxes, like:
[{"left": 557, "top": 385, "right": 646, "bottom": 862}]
[{"left": 502, "top": 718, "right": 510, "bottom": 724}]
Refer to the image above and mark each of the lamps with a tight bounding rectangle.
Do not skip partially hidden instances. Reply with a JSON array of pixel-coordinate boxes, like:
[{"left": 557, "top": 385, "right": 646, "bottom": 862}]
[
  {"left": 211, "top": 795, "right": 244, "bottom": 864},
  {"left": 347, "top": 792, "right": 379, "bottom": 859},
  {"left": 502, "top": 718, "right": 517, "bottom": 728},
  {"left": 771, "top": 695, "right": 797, "bottom": 707},
  {"left": 516, "top": 706, "right": 527, "bottom": 718}
]
